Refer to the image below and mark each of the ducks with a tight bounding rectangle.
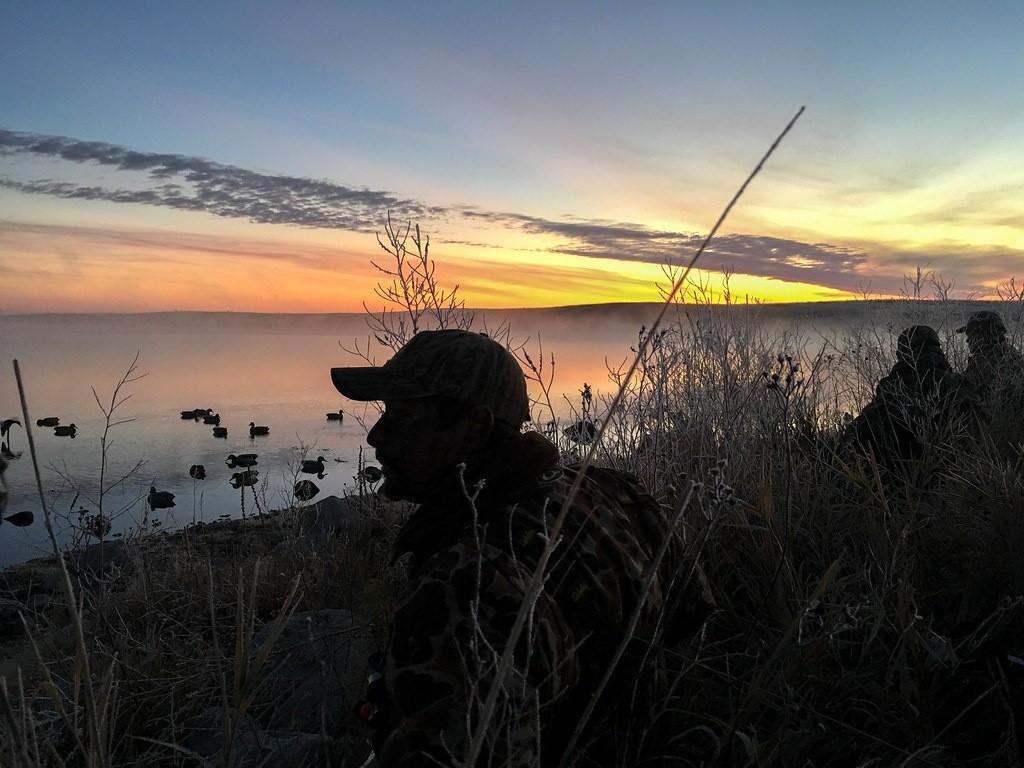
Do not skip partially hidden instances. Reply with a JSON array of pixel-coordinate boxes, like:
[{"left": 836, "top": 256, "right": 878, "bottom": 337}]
[{"left": 35, "top": 407, "right": 383, "bottom": 502}]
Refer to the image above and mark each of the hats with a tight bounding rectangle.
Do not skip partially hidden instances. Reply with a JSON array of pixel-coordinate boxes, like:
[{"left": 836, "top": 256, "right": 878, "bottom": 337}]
[
  {"left": 956, "top": 311, "right": 1005, "bottom": 334},
  {"left": 331, "top": 329, "right": 531, "bottom": 429},
  {"left": 898, "top": 326, "right": 939, "bottom": 351}
]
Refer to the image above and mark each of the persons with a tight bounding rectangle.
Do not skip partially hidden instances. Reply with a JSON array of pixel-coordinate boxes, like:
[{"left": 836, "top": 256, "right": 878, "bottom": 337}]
[
  {"left": 331, "top": 329, "right": 743, "bottom": 768},
  {"left": 842, "top": 310, "right": 1023, "bottom": 480}
]
